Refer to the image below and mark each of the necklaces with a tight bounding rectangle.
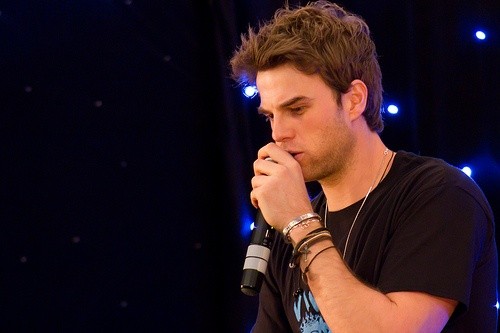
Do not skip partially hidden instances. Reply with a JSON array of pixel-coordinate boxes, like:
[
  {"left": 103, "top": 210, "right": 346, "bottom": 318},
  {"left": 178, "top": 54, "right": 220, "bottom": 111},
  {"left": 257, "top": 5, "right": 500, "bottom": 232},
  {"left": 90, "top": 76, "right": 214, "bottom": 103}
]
[{"left": 324, "top": 148, "right": 395, "bottom": 259}]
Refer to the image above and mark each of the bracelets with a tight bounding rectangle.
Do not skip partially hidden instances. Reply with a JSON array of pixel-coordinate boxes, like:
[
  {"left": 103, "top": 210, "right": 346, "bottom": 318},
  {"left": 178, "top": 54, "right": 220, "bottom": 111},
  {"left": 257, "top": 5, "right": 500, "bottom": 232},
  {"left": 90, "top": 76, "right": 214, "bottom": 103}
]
[{"left": 282, "top": 213, "right": 336, "bottom": 284}]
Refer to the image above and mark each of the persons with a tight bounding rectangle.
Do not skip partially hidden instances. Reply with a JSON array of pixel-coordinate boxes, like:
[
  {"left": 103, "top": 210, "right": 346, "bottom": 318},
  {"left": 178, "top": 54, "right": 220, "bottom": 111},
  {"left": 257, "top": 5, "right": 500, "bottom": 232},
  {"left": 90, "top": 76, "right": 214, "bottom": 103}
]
[{"left": 229, "top": 0, "right": 498, "bottom": 333}]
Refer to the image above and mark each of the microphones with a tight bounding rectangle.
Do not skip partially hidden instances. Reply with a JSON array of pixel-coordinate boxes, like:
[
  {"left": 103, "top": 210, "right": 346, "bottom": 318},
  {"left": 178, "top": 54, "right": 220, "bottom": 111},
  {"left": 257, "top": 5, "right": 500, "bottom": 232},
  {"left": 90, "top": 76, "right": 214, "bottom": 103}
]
[{"left": 240, "top": 158, "right": 278, "bottom": 297}]
